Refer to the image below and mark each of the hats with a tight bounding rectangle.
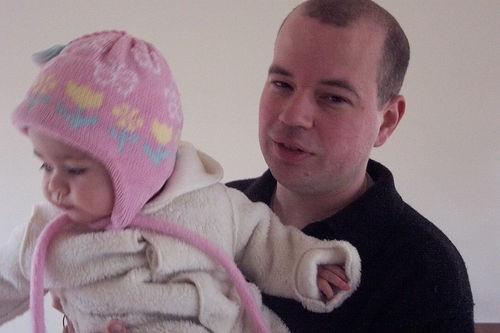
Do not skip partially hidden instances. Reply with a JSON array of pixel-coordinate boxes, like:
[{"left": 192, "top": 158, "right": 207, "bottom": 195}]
[{"left": 11, "top": 29, "right": 184, "bottom": 229}]
